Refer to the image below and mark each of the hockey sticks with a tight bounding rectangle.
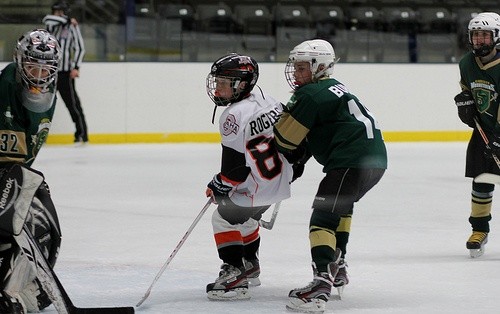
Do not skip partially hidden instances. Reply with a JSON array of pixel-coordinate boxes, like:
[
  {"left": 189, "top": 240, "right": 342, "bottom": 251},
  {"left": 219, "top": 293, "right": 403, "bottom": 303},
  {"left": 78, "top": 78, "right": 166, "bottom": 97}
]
[
  {"left": 22, "top": 224, "right": 136, "bottom": 314},
  {"left": 476, "top": 122, "right": 500, "bottom": 169},
  {"left": 137, "top": 196, "right": 214, "bottom": 306},
  {"left": 260, "top": 202, "right": 281, "bottom": 230}
]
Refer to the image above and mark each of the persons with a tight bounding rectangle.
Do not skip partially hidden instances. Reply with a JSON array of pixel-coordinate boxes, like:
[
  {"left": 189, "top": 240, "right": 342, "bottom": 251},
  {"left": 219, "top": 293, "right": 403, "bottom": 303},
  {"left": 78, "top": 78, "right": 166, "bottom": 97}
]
[
  {"left": 0, "top": 30, "right": 63, "bottom": 314},
  {"left": 46, "top": 5, "right": 89, "bottom": 149},
  {"left": 206, "top": 52, "right": 306, "bottom": 300},
  {"left": 273, "top": 38, "right": 387, "bottom": 314},
  {"left": 454, "top": 12, "right": 500, "bottom": 257}
]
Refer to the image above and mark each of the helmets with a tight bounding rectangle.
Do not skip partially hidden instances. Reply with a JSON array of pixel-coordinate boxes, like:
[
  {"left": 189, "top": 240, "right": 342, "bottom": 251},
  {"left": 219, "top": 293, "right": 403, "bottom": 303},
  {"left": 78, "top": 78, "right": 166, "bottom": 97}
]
[
  {"left": 285, "top": 39, "right": 335, "bottom": 90},
  {"left": 467, "top": 12, "right": 500, "bottom": 56},
  {"left": 13, "top": 29, "right": 61, "bottom": 93},
  {"left": 206, "top": 53, "right": 259, "bottom": 105},
  {"left": 52, "top": 2, "right": 66, "bottom": 10}
]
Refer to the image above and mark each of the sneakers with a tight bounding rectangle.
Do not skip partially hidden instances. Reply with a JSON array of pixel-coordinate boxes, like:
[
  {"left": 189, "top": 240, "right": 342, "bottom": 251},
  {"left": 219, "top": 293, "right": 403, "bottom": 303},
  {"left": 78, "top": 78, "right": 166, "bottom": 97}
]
[
  {"left": 334, "top": 259, "right": 349, "bottom": 300},
  {"left": 286, "top": 261, "right": 339, "bottom": 314},
  {"left": 244, "top": 259, "right": 262, "bottom": 286},
  {"left": 206, "top": 263, "right": 249, "bottom": 300},
  {"left": 466, "top": 231, "right": 488, "bottom": 258}
]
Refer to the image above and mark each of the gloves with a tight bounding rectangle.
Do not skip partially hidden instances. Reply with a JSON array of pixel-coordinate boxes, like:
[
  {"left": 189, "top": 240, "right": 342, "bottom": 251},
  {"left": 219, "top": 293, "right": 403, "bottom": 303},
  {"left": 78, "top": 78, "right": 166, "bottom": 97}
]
[
  {"left": 484, "top": 137, "right": 500, "bottom": 159},
  {"left": 206, "top": 174, "right": 232, "bottom": 204},
  {"left": 454, "top": 90, "right": 479, "bottom": 128}
]
